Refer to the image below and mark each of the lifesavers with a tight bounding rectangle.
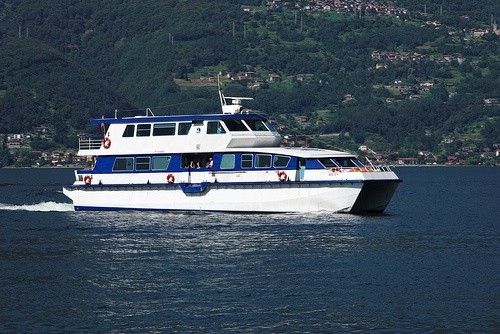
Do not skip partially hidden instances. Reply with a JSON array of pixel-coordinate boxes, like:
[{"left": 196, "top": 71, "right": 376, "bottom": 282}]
[
  {"left": 83, "top": 175, "right": 91, "bottom": 184},
  {"left": 279, "top": 171, "right": 287, "bottom": 181},
  {"left": 102, "top": 137, "right": 111, "bottom": 149},
  {"left": 167, "top": 174, "right": 174, "bottom": 183}
]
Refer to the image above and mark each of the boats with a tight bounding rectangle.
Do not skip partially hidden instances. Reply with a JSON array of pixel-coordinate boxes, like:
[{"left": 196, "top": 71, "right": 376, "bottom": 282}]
[{"left": 62, "top": 76, "right": 401, "bottom": 216}]
